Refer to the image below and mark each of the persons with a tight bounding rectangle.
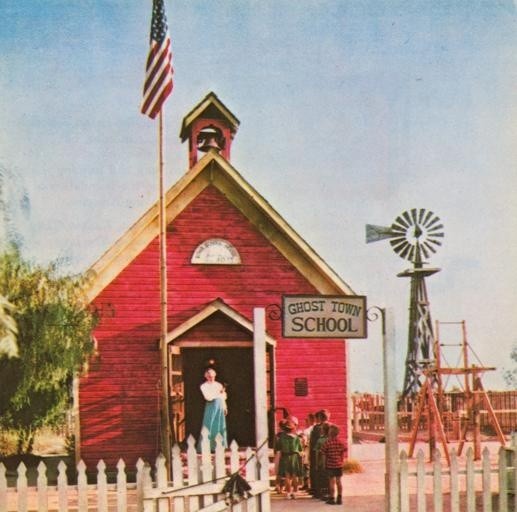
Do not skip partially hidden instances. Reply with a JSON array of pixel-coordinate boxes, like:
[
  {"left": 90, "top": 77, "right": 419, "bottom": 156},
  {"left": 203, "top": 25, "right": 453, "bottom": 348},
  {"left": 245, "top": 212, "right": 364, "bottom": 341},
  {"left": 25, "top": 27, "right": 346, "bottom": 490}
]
[
  {"left": 273, "top": 409, "right": 348, "bottom": 505},
  {"left": 200, "top": 367, "right": 229, "bottom": 453}
]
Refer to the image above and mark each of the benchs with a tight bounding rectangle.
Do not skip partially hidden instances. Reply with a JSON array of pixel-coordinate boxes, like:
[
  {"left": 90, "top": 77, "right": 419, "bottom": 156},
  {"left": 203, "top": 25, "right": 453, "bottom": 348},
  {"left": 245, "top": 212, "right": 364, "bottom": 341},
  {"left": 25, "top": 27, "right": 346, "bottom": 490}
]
[{"left": 141, "top": 0, "right": 173, "bottom": 119}]
